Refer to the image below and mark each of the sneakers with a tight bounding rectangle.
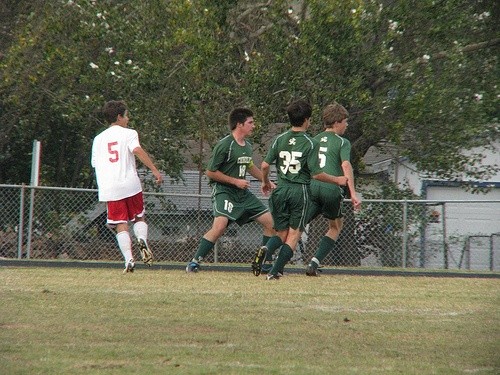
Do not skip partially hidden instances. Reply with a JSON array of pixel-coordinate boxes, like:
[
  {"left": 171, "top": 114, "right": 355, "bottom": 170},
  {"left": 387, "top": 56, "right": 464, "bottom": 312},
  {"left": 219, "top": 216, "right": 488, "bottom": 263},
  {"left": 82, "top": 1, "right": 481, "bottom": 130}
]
[
  {"left": 253, "top": 245, "right": 268, "bottom": 277},
  {"left": 306, "top": 261, "right": 318, "bottom": 276},
  {"left": 136, "top": 238, "right": 153, "bottom": 268},
  {"left": 266, "top": 274, "right": 279, "bottom": 280},
  {"left": 186, "top": 256, "right": 204, "bottom": 273},
  {"left": 123, "top": 258, "right": 136, "bottom": 274},
  {"left": 261, "top": 264, "right": 283, "bottom": 277}
]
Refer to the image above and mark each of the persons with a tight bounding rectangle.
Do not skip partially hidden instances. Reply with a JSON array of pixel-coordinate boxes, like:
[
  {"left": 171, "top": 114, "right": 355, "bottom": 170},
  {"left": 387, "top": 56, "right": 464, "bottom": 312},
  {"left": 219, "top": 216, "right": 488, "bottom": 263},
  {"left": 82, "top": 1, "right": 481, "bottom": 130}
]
[
  {"left": 91, "top": 100, "right": 163, "bottom": 272},
  {"left": 252, "top": 99, "right": 348, "bottom": 280},
  {"left": 186, "top": 107, "right": 281, "bottom": 274},
  {"left": 304, "top": 103, "right": 362, "bottom": 276}
]
